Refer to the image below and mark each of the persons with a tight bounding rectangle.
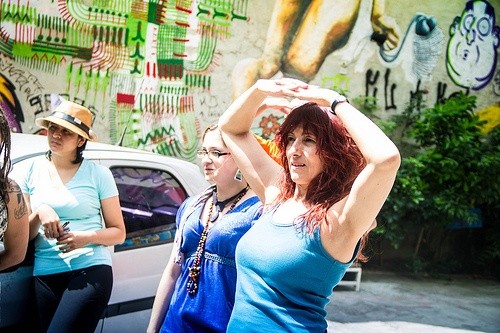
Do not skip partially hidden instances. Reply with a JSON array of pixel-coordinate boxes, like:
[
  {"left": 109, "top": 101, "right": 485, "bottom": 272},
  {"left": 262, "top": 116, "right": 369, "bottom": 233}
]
[
  {"left": 218, "top": 78, "right": 401, "bottom": 333},
  {"left": 0, "top": 107, "right": 29, "bottom": 275},
  {"left": 147, "top": 123, "right": 264, "bottom": 333},
  {"left": 20, "top": 101, "right": 126, "bottom": 333}
]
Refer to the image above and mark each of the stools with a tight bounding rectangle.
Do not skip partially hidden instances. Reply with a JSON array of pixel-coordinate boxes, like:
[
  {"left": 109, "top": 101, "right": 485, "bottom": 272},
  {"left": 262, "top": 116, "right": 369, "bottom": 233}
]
[{"left": 335, "top": 266, "right": 363, "bottom": 291}]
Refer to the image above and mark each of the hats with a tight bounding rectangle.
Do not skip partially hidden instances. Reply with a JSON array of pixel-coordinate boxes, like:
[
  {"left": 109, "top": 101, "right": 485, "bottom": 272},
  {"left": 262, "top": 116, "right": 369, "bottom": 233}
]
[{"left": 35, "top": 101, "right": 97, "bottom": 141}]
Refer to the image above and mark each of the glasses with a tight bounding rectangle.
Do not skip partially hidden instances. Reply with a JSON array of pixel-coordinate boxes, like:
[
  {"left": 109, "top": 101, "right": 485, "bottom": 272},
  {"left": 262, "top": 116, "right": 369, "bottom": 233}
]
[{"left": 196, "top": 150, "right": 231, "bottom": 159}]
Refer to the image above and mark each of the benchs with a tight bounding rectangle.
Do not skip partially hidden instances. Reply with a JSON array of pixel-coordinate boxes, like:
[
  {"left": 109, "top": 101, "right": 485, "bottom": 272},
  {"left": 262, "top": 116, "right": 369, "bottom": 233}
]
[{"left": 115, "top": 183, "right": 179, "bottom": 235}]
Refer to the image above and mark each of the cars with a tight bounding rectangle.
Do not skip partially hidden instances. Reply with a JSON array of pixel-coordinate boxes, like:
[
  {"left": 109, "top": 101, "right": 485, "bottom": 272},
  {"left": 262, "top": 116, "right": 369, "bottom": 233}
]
[{"left": 0, "top": 132, "right": 213, "bottom": 333}]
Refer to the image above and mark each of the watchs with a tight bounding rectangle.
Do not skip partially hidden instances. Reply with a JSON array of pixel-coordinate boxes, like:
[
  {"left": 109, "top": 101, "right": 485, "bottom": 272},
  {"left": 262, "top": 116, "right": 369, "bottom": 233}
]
[{"left": 329, "top": 96, "right": 349, "bottom": 115}]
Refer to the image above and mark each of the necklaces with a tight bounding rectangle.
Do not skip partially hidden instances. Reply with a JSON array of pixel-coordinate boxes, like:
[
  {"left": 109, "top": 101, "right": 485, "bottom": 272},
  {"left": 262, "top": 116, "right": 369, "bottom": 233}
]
[
  {"left": 210, "top": 186, "right": 247, "bottom": 222},
  {"left": 186, "top": 184, "right": 251, "bottom": 294}
]
